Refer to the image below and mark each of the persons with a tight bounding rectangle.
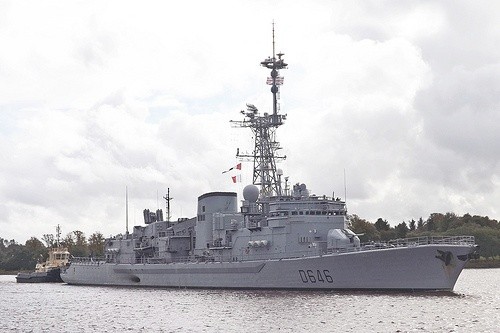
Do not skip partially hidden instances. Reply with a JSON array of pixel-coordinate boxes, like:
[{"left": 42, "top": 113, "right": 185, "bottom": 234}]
[{"left": 232, "top": 257, "right": 238, "bottom": 262}]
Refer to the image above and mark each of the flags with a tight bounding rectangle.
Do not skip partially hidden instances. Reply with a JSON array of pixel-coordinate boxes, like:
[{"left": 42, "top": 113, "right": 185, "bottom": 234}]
[
  {"left": 266, "top": 77, "right": 284, "bottom": 85},
  {"left": 223, "top": 163, "right": 241, "bottom": 173},
  {"left": 232, "top": 174, "right": 240, "bottom": 184}
]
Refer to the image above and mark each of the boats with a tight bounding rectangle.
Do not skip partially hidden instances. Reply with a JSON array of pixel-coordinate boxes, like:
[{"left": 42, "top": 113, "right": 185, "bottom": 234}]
[
  {"left": 14, "top": 246, "right": 74, "bottom": 283},
  {"left": 60, "top": 20, "right": 480, "bottom": 295}
]
